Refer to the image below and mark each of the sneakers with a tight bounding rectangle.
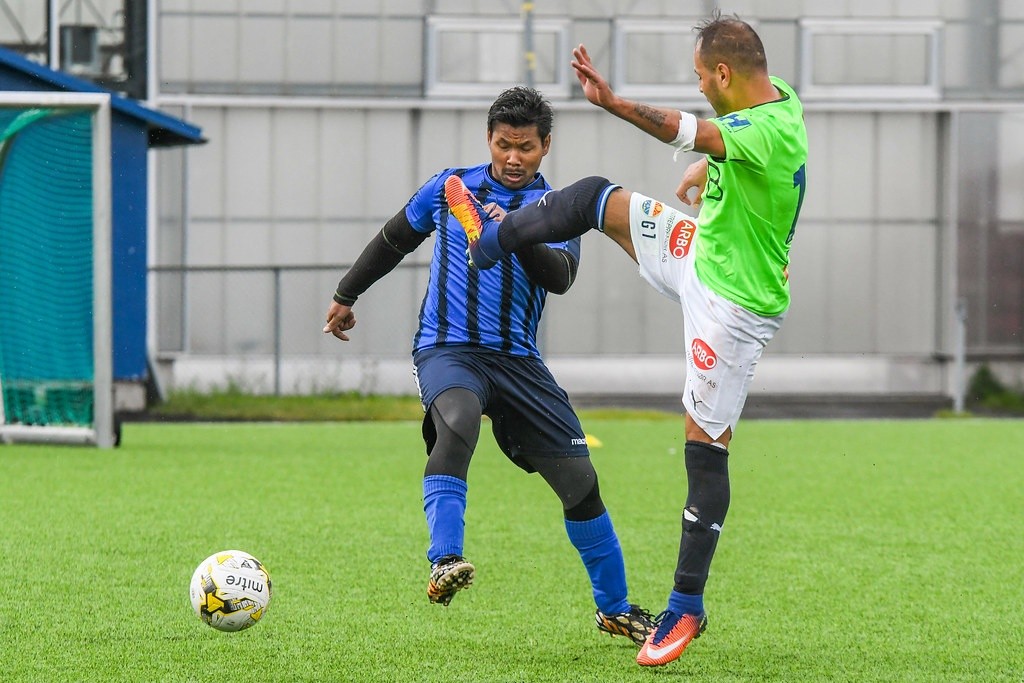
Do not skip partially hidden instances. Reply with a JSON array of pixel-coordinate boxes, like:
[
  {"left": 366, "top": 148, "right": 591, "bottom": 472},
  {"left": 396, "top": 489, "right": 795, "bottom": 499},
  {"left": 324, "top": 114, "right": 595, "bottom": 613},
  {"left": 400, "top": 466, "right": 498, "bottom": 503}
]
[
  {"left": 636, "top": 611, "right": 708, "bottom": 668},
  {"left": 595, "top": 604, "right": 658, "bottom": 648},
  {"left": 426, "top": 556, "right": 475, "bottom": 607},
  {"left": 444, "top": 175, "right": 499, "bottom": 270}
]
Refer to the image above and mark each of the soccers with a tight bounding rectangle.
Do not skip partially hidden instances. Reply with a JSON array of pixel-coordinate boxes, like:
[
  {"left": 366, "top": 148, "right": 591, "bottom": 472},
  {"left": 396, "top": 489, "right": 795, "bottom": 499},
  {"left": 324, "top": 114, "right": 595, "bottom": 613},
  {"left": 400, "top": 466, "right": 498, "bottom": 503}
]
[{"left": 189, "top": 550, "right": 272, "bottom": 634}]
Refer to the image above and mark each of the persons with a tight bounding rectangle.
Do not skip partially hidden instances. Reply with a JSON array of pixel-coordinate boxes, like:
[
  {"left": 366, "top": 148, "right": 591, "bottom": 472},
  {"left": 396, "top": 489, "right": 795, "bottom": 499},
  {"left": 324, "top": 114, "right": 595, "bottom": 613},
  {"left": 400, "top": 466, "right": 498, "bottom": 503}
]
[
  {"left": 443, "top": 7, "right": 809, "bottom": 670},
  {"left": 322, "top": 82, "right": 661, "bottom": 647}
]
